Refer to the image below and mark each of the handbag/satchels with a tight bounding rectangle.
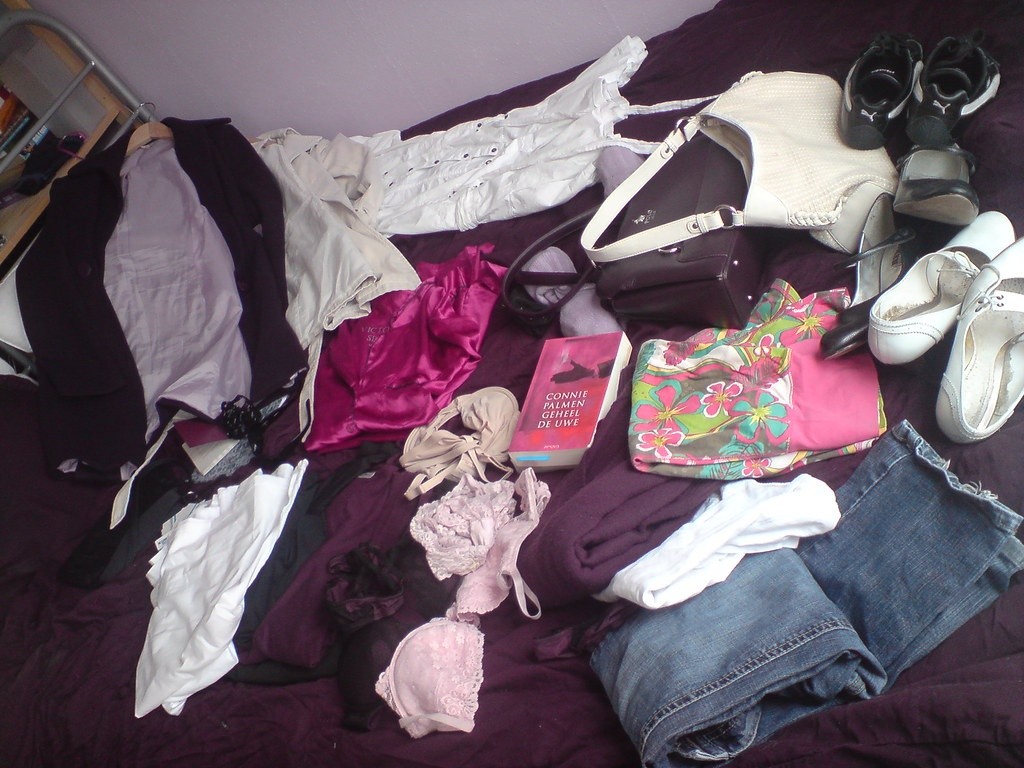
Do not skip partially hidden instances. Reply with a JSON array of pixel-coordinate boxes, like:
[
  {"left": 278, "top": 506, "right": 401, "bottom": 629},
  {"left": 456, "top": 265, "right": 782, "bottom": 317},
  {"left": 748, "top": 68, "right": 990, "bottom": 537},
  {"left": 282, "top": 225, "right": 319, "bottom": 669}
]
[
  {"left": 502, "top": 132, "right": 767, "bottom": 331},
  {"left": 580, "top": 69, "right": 903, "bottom": 257}
]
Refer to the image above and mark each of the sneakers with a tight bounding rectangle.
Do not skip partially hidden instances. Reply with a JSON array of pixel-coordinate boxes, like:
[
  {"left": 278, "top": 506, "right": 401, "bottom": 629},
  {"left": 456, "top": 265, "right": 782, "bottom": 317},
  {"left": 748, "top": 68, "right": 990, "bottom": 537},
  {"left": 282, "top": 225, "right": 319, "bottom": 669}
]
[
  {"left": 837, "top": 31, "right": 925, "bottom": 150},
  {"left": 905, "top": 28, "right": 1002, "bottom": 146}
]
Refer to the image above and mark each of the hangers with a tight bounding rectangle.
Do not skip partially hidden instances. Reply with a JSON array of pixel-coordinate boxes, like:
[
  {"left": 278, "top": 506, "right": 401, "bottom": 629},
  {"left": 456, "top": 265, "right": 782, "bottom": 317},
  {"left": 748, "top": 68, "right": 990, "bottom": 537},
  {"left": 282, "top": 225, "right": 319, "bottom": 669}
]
[{"left": 12, "top": 104, "right": 283, "bottom": 168}]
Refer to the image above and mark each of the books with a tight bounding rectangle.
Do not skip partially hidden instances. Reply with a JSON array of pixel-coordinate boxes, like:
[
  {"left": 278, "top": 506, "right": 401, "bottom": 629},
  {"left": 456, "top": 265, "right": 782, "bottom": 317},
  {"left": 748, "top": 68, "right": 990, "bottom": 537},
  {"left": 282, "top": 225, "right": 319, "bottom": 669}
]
[
  {"left": 507, "top": 332, "right": 632, "bottom": 473},
  {"left": 0, "top": 85, "right": 49, "bottom": 174}
]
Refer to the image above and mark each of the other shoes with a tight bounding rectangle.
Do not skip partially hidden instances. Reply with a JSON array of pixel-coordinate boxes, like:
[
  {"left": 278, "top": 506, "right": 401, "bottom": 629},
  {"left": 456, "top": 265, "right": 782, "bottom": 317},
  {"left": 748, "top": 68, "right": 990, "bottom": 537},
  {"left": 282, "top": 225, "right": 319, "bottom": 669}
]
[
  {"left": 818, "top": 193, "right": 921, "bottom": 361},
  {"left": 869, "top": 208, "right": 1016, "bottom": 368},
  {"left": 936, "top": 237, "right": 1024, "bottom": 445},
  {"left": 893, "top": 140, "right": 980, "bottom": 226}
]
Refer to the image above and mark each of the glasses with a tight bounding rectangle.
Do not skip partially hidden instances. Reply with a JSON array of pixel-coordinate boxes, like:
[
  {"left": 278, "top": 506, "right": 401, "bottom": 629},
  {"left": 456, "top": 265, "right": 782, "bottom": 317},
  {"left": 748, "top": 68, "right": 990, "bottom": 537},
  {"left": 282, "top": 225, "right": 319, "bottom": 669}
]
[{"left": 246, "top": 379, "right": 311, "bottom": 469}]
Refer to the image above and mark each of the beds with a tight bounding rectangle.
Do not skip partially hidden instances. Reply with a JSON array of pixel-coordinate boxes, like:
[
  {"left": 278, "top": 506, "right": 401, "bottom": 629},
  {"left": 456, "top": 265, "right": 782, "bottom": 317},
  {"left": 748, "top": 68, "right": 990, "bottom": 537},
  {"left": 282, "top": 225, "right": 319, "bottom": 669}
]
[{"left": 0, "top": 0, "right": 1024, "bottom": 768}]
